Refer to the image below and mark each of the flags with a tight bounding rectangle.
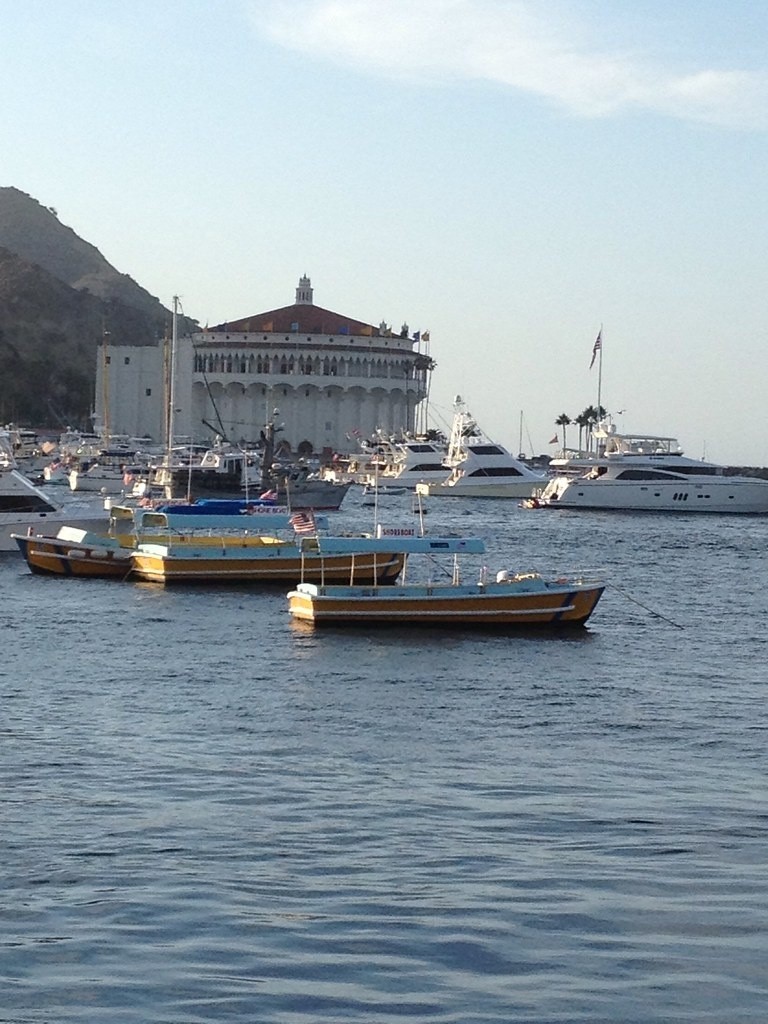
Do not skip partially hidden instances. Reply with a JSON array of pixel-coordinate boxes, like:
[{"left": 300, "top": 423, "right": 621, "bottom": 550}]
[
  {"left": 289, "top": 513, "right": 314, "bottom": 533},
  {"left": 203, "top": 323, "right": 430, "bottom": 342},
  {"left": 589, "top": 330, "right": 601, "bottom": 368}
]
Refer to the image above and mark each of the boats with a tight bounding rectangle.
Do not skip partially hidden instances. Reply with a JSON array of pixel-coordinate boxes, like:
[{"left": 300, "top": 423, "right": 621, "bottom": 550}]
[
  {"left": 285, "top": 535, "right": 606, "bottom": 627},
  {"left": 416, "top": 397, "right": 549, "bottom": 497},
  {"left": 531, "top": 429, "right": 768, "bottom": 514},
  {"left": 9, "top": 507, "right": 138, "bottom": 574},
  {"left": 127, "top": 511, "right": 414, "bottom": 587},
  {"left": 43, "top": 293, "right": 350, "bottom": 522},
  {"left": 326, "top": 431, "right": 454, "bottom": 486},
  {"left": 0, "top": 423, "right": 62, "bottom": 517}
]
